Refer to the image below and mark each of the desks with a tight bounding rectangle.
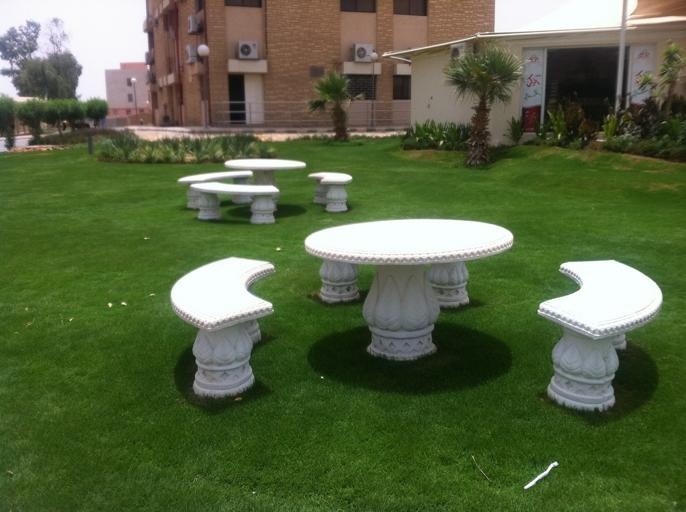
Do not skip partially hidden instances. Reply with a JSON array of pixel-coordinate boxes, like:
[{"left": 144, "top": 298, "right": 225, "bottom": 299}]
[
  {"left": 296, "top": 218, "right": 516, "bottom": 364},
  {"left": 222, "top": 158, "right": 310, "bottom": 212}
]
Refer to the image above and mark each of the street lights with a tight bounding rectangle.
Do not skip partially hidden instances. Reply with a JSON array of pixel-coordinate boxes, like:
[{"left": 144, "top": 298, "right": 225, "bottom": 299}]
[
  {"left": 129, "top": 77, "right": 139, "bottom": 116},
  {"left": 195, "top": 43, "right": 211, "bottom": 130}
]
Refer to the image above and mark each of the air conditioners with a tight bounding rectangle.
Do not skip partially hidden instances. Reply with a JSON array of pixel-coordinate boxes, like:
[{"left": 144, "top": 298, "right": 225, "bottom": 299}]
[
  {"left": 182, "top": 42, "right": 201, "bottom": 67},
  {"left": 236, "top": 39, "right": 258, "bottom": 63},
  {"left": 448, "top": 40, "right": 475, "bottom": 66},
  {"left": 351, "top": 42, "right": 376, "bottom": 66},
  {"left": 185, "top": 13, "right": 204, "bottom": 38}
]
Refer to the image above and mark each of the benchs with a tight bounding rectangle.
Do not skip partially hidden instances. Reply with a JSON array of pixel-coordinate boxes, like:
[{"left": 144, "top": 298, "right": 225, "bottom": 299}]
[
  {"left": 168, "top": 254, "right": 277, "bottom": 402},
  {"left": 537, "top": 259, "right": 664, "bottom": 417},
  {"left": 306, "top": 172, "right": 353, "bottom": 214},
  {"left": 186, "top": 182, "right": 280, "bottom": 227},
  {"left": 177, "top": 170, "right": 256, "bottom": 207}
]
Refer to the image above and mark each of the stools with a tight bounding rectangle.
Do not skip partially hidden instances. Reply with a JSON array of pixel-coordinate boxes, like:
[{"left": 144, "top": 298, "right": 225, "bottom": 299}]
[
  {"left": 427, "top": 261, "right": 470, "bottom": 310},
  {"left": 317, "top": 256, "right": 360, "bottom": 304}
]
